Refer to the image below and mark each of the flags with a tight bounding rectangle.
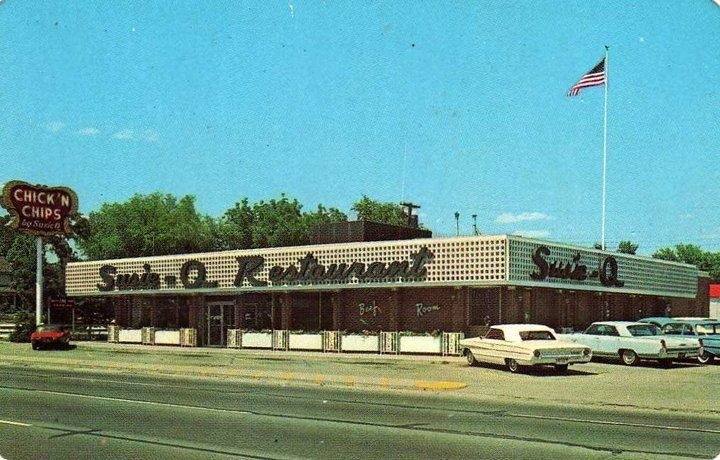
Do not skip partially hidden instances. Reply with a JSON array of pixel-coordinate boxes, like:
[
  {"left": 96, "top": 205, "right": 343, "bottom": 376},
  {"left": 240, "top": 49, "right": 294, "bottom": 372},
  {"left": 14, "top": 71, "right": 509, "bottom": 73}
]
[{"left": 567, "top": 56, "right": 606, "bottom": 97}]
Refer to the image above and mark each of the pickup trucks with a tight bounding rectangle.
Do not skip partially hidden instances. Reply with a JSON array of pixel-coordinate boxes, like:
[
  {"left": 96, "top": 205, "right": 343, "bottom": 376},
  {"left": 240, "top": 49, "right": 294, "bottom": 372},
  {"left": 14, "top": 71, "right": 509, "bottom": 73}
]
[{"left": 672, "top": 317, "right": 720, "bottom": 364}]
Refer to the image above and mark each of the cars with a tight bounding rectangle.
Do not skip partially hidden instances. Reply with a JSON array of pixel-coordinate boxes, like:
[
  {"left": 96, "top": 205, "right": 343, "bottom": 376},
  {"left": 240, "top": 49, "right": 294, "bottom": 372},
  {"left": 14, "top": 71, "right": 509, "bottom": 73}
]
[
  {"left": 459, "top": 323, "right": 593, "bottom": 373},
  {"left": 31, "top": 323, "right": 71, "bottom": 351},
  {"left": 638, "top": 317, "right": 699, "bottom": 363},
  {"left": 562, "top": 320, "right": 704, "bottom": 369}
]
